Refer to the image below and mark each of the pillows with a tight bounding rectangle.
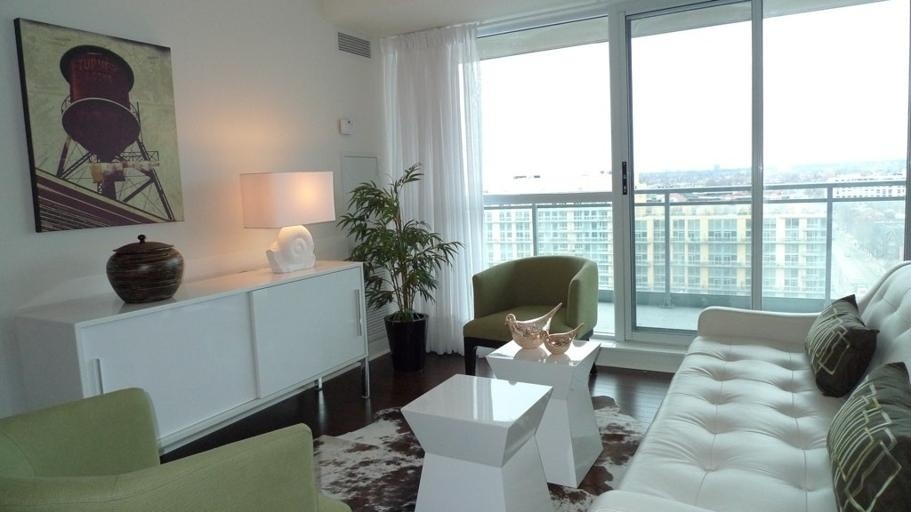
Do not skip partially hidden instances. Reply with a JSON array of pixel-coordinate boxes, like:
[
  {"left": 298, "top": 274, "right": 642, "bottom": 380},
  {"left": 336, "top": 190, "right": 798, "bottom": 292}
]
[
  {"left": 826, "top": 362, "right": 911, "bottom": 512},
  {"left": 805, "top": 294, "right": 880, "bottom": 397}
]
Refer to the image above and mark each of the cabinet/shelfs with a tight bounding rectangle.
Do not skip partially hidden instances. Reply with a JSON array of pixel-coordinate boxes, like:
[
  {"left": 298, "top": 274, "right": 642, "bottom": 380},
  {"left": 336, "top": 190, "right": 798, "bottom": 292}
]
[{"left": 15, "top": 261, "right": 370, "bottom": 458}]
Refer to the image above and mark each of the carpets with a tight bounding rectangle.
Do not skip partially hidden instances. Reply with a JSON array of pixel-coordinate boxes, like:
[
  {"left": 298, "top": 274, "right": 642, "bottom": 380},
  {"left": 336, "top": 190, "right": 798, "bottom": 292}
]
[{"left": 314, "top": 395, "right": 647, "bottom": 512}]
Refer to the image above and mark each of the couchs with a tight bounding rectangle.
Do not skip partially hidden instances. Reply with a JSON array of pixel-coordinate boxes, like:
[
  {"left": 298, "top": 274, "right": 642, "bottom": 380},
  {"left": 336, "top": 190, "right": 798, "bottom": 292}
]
[{"left": 585, "top": 261, "right": 911, "bottom": 512}]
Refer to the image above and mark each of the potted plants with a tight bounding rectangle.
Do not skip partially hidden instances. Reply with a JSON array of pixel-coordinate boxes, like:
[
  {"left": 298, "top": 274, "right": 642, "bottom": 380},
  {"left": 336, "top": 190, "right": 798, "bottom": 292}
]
[{"left": 336, "top": 164, "right": 464, "bottom": 374}]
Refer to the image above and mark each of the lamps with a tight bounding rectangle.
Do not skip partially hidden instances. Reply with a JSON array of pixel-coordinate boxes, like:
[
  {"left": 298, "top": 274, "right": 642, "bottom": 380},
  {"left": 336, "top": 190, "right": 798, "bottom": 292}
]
[{"left": 238, "top": 171, "right": 336, "bottom": 272}]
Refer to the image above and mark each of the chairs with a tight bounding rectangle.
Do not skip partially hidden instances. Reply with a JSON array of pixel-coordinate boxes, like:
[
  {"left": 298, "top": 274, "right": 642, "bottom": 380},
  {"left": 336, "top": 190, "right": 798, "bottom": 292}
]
[
  {"left": 464, "top": 255, "right": 598, "bottom": 376},
  {"left": 0, "top": 388, "right": 352, "bottom": 512}
]
